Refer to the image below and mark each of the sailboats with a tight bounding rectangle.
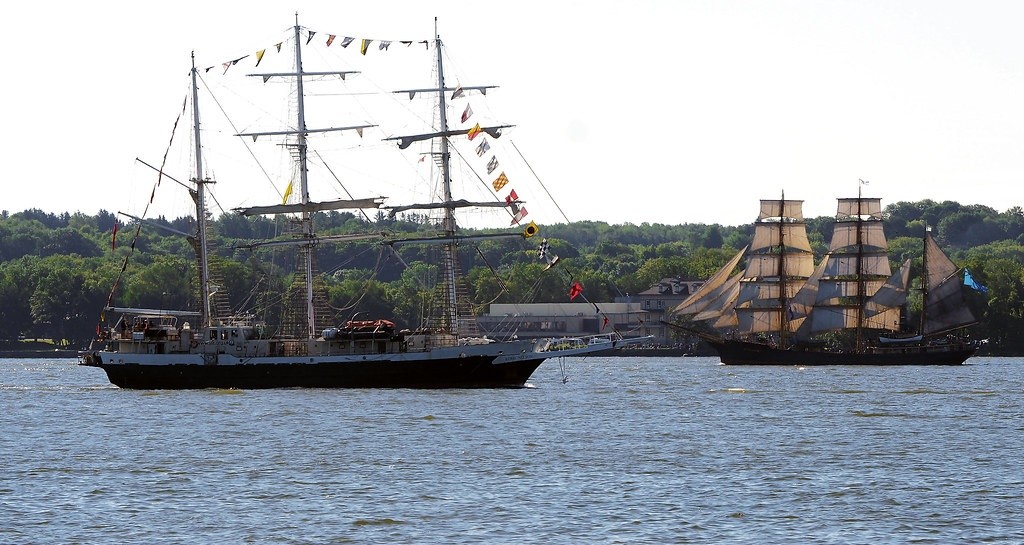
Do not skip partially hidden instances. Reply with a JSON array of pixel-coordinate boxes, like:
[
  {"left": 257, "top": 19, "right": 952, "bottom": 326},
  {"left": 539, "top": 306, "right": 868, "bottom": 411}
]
[
  {"left": 658, "top": 186, "right": 980, "bottom": 366},
  {"left": 76, "top": 13, "right": 655, "bottom": 389}
]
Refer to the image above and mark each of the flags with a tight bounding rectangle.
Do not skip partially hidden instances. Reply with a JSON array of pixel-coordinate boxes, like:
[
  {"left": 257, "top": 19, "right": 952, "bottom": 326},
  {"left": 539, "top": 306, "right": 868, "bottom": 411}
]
[
  {"left": 306, "top": 31, "right": 315, "bottom": 45},
  {"left": 341, "top": 36, "right": 355, "bottom": 48},
  {"left": 505, "top": 189, "right": 519, "bottom": 205},
  {"left": 568, "top": 281, "right": 584, "bottom": 301},
  {"left": 475, "top": 138, "right": 490, "bottom": 157},
  {"left": 461, "top": 102, "right": 472, "bottom": 123},
  {"left": 379, "top": 41, "right": 392, "bottom": 51},
  {"left": 603, "top": 315, "right": 609, "bottom": 330},
  {"left": 524, "top": 220, "right": 539, "bottom": 240},
  {"left": 964, "top": 267, "right": 988, "bottom": 293},
  {"left": 360, "top": 39, "right": 374, "bottom": 55},
  {"left": 88, "top": 96, "right": 187, "bottom": 352},
  {"left": 486, "top": 155, "right": 499, "bottom": 175},
  {"left": 492, "top": 171, "right": 509, "bottom": 192},
  {"left": 468, "top": 123, "right": 481, "bottom": 141},
  {"left": 536, "top": 238, "right": 551, "bottom": 259},
  {"left": 451, "top": 84, "right": 462, "bottom": 100},
  {"left": 326, "top": 35, "right": 336, "bottom": 47},
  {"left": 205, "top": 42, "right": 281, "bottom": 75},
  {"left": 511, "top": 206, "right": 529, "bottom": 226}
]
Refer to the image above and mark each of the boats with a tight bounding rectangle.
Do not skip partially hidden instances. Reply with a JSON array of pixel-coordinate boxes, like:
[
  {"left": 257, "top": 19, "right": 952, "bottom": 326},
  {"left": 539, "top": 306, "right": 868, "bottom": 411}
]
[{"left": 79, "top": 311, "right": 179, "bottom": 366}]
